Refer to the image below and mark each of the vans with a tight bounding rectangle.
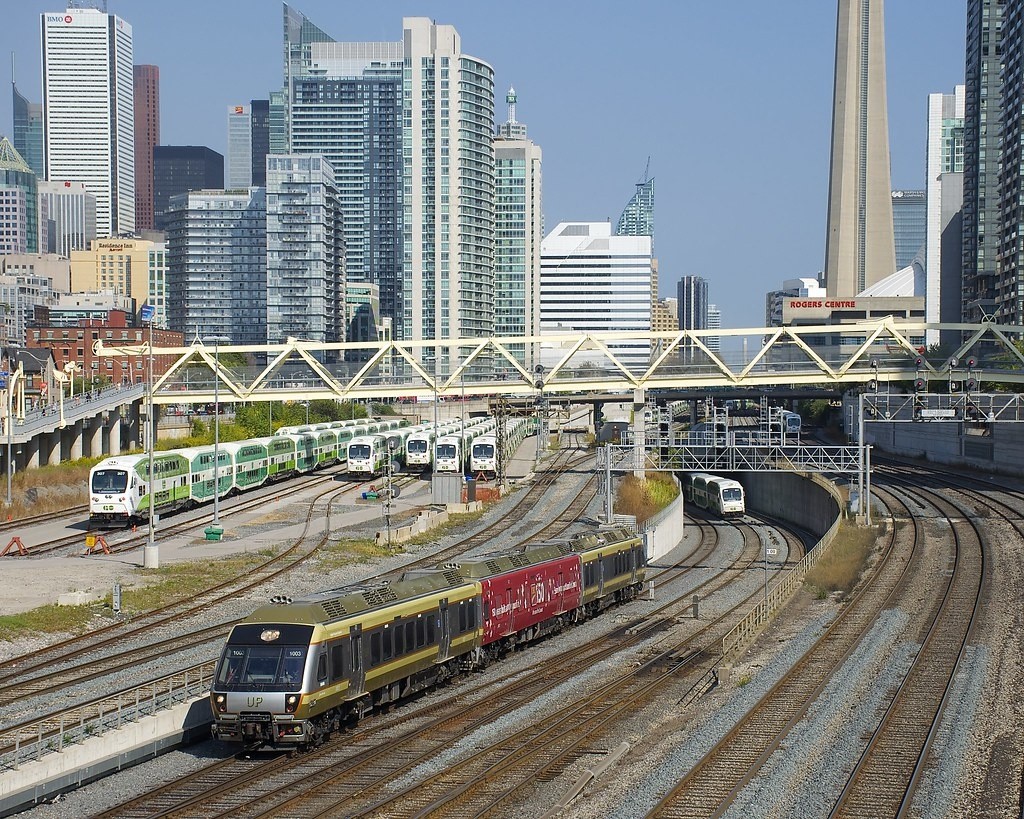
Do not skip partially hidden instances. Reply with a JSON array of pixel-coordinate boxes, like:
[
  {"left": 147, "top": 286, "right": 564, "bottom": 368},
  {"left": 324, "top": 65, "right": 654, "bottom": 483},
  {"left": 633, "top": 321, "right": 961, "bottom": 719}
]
[{"left": 501, "top": 394, "right": 520, "bottom": 398}]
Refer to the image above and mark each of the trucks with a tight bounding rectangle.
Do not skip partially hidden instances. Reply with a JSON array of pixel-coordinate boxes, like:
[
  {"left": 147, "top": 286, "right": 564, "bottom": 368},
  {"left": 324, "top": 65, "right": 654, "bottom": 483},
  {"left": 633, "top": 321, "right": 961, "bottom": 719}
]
[{"left": 417, "top": 396, "right": 445, "bottom": 403}]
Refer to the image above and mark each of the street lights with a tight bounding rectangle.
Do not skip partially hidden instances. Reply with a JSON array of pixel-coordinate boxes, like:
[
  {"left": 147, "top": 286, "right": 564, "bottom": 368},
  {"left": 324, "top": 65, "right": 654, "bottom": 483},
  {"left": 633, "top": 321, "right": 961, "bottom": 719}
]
[
  {"left": 3, "top": 357, "right": 15, "bottom": 501},
  {"left": 104, "top": 358, "right": 129, "bottom": 387},
  {"left": 291, "top": 370, "right": 302, "bottom": 388},
  {"left": 228, "top": 369, "right": 245, "bottom": 387},
  {"left": 96, "top": 342, "right": 155, "bottom": 542},
  {"left": 204, "top": 334, "right": 232, "bottom": 524},
  {"left": 166, "top": 364, "right": 202, "bottom": 389},
  {"left": 17, "top": 349, "right": 50, "bottom": 410},
  {"left": 48, "top": 360, "right": 69, "bottom": 409},
  {"left": 457, "top": 364, "right": 472, "bottom": 475}
]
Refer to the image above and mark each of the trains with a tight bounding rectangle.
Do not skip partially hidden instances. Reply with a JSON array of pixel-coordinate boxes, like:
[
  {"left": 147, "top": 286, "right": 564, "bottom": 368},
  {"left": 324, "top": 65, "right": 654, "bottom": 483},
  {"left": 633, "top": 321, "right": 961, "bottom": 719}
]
[
  {"left": 644, "top": 400, "right": 688, "bottom": 423},
  {"left": 682, "top": 472, "right": 746, "bottom": 517},
  {"left": 211, "top": 524, "right": 651, "bottom": 761},
  {"left": 87, "top": 417, "right": 546, "bottom": 526},
  {"left": 771, "top": 404, "right": 801, "bottom": 434},
  {"left": 726, "top": 400, "right": 738, "bottom": 410}
]
